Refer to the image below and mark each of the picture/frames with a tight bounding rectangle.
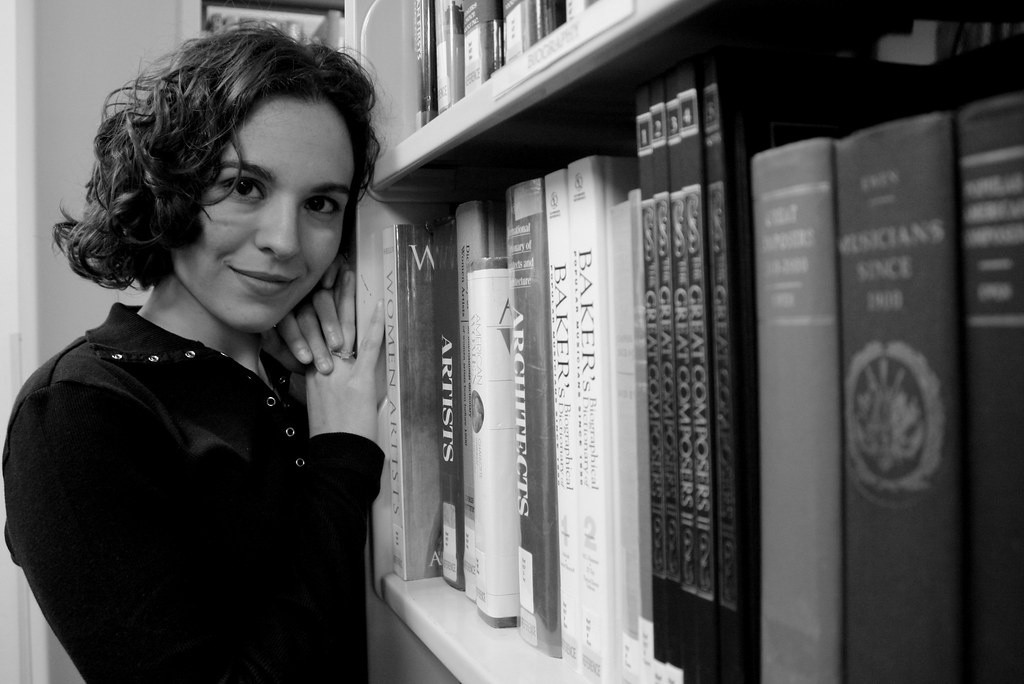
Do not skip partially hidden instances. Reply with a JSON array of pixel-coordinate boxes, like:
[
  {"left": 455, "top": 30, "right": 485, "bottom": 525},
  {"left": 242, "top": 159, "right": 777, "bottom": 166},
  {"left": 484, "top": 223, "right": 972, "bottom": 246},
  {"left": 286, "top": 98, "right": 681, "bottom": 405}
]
[{"left": 202, "top": 0, "right": 345, "bottom": 47}]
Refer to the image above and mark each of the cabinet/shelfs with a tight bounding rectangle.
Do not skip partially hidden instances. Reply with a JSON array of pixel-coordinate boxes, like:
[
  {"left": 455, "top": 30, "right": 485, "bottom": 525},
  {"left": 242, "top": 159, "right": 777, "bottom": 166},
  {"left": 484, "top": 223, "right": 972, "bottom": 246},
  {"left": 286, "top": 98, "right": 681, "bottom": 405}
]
[{"left": 345, "top": 0, "right": 1024, "bottom": 684}]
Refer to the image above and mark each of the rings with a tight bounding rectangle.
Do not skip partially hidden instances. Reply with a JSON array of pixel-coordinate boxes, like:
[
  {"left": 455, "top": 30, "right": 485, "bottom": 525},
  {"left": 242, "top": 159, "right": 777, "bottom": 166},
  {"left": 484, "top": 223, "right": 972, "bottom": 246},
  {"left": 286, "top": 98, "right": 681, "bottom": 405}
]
[{"left": 331, "top": 349, "right": 356, "bottom": 359}]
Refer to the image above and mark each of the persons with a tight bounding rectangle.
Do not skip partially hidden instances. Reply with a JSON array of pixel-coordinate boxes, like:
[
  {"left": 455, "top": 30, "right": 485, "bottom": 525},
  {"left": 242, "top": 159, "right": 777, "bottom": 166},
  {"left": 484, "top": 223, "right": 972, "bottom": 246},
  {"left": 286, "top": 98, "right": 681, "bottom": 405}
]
[{"left": 0, "top": 18, "right": 385, "bottom": 684}]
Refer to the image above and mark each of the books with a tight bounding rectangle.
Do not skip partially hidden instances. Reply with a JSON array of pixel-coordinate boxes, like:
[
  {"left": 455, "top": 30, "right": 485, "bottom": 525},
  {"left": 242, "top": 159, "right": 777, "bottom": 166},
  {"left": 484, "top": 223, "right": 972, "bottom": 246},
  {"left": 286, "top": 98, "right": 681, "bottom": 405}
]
[
  {"left": 210, "top": 11, "right": 344, "bottom": 47},
  {"left": 378, "top": 0, "right": 1024, "bottom": 684}
]
